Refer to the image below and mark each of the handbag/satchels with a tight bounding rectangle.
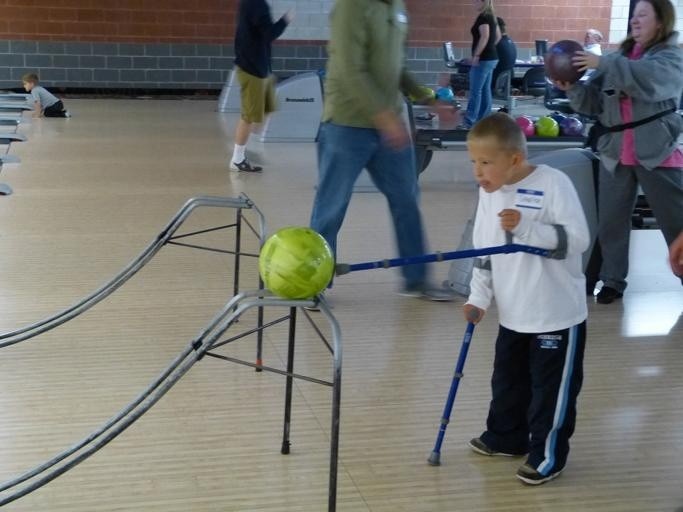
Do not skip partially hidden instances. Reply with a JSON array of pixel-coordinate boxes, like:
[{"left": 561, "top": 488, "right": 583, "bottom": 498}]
[{"left": 582, "top": 121, "right": 610, "bottom": 152}]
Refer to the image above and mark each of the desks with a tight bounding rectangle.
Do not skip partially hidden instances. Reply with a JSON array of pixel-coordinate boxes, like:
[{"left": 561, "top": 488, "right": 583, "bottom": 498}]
[{"left": 514, "top": 61, "right": 545, "bottom": 67}]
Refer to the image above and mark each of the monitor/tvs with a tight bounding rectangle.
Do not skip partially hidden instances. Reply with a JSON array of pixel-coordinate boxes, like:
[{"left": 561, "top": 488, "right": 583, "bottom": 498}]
[
  {"left": 537, "top": 40, "right": 548, "bottom": 56},
  {"left": 443, "top": 41, "right": 453, "bottom": 61}
]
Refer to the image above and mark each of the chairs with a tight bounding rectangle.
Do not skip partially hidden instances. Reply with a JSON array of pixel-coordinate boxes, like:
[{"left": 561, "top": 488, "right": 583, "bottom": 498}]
[
  {"left": 522, "top": 67, "right": 549, "bottom": 95},
  {"left": 443, "top": 40, "right": 470, "bottom": 90}
]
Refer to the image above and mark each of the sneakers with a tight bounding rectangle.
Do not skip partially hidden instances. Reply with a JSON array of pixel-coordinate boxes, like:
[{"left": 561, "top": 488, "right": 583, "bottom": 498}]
[
  {"left": 230, "top": 160, "right": 263, "bottom": 172},
  {"left": 457, "top": 122, "right": 467, "bottom": 128},
  {"left": 597, "top": 287, "right": 622, "bottom": 304},
  {"left": 469, "top": 439, "right": 515, "bottom": 456},
  {"left": 516, "top": 464, "right": 567, "bottom": 485},
  {"left": 401, "top": 286, "right": 455, "bottom": 300}
]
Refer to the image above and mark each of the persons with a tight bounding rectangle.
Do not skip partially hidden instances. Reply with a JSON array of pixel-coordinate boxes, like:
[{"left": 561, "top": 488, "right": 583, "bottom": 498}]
[
  {"left": 493, "top": 17, "right": 517, "bottom": 100},
  {"left": 551, "top": 0, "right": 683, "bottom": 304},
  {"left": 463, "top": 112, "right": 590, "bottom": 485},
  {"left": 457, "top": 1, "right": 502, "bottom": 131},
  {"left": 549, "top": 29, "right": 603, "bottom": 98},
  {"left": 23, "top": 72, "right": 72, "bottom": 118},
  {"left": 669, "top": 230, "right": 683, "bottom": 276},
  {"left": 228, "top": 0, "right": 294, "bottom": 173},
  {"left": 305, "top": 0, "right": 457, "bottom": 313}
]
